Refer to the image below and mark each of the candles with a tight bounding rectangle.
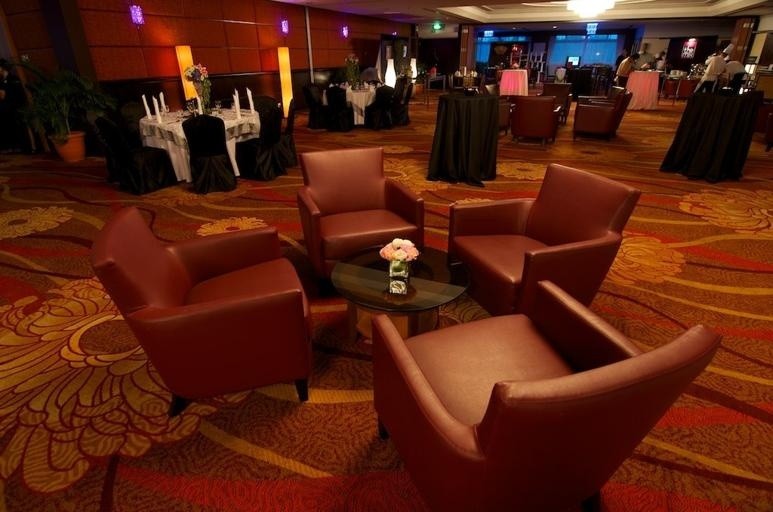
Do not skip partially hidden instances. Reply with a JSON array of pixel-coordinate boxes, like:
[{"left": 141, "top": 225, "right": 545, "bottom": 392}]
[{"left": 455, "top": 66, "right": 479, "bottom": 96}]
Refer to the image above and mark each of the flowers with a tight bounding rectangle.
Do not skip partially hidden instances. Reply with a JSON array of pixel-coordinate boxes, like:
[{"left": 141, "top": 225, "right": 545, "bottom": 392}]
[
  {"left": 345, "top": 54, "right": 361, "bottom": 83},
  {"left": 379, "top": 239, "right": 418, "bottom": 262},
  {"left": 184, "top": 65, "right": 208, "bottom": 82}
]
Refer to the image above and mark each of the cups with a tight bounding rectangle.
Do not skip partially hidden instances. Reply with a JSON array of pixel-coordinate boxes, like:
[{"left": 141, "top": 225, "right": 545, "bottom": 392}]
[
  {"left": 161, "top": 100, "right": 236, "bottom": 123},
  {"left": 335, "top": 81, "right": 380, "bottom": 91}
]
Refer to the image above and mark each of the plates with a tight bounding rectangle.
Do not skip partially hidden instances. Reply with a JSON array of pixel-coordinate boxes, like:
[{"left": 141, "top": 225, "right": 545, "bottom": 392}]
[{"left": 670, "top": 70, "right": 687, "bottom": 79}]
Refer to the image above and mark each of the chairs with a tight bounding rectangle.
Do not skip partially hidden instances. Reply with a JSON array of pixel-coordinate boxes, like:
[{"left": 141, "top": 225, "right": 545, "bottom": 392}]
[
  {"left": 654, "top": 70, "right": 716, "bottom": 108},
  {"left": 96, "top": 85, "right": 298, "bottom": 194},
  {"left": 91, "top": 205, "right": 315, "bottom": 417},
  {"left": 305, "top": 75, "right": 413, "bottom": 133},
  {"left": 370, "top": 281, "right": 722, "bottom": 508},
  {"left": 442, "top": 164, "right": 641, "bottom": 320},
  {"left": 297, "top": 144, "right": 426, "bottom": 294},
  {"left": 480, "top": 63, "right": 633, "bottom": 146}
]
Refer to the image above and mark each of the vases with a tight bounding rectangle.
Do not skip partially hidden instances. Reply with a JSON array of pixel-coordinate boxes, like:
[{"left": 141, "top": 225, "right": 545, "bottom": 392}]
[{"left": 388, "top": 259, "right": 410, "bottom": 277}]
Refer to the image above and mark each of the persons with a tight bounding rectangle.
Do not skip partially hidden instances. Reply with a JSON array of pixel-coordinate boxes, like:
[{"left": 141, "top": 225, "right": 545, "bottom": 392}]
[
  {"left": 615, "top": 47, "right": 632, "bottom": 72},
  {"left": 693, "top": 49, "right": 731, "bottom": 93},
  {"left": 722, "top": 59, "right": 748, "bottom": 94},
  {"left": 655, "top": 51, "right": 665, "bottom": 69},
  {"left": 633, "top": 43, "right": 652, "bottom": 70},
  {"left": 616, "top": 53, "right": 640, "bottom": 87}
]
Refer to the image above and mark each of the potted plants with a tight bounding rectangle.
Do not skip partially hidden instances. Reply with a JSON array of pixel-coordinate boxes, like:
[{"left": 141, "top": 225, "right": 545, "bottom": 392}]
[{"left": 14, "top": 62, "right": 116, "bottom": 162}]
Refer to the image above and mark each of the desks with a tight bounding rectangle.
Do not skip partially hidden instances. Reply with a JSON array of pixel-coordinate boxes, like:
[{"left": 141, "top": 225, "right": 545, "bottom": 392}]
[
  {"left": 687, "top": 90, "right": 764, "bottom": 185},
  {"left": 439, "top": 93, "right": 498, "bottom": 186}
]
